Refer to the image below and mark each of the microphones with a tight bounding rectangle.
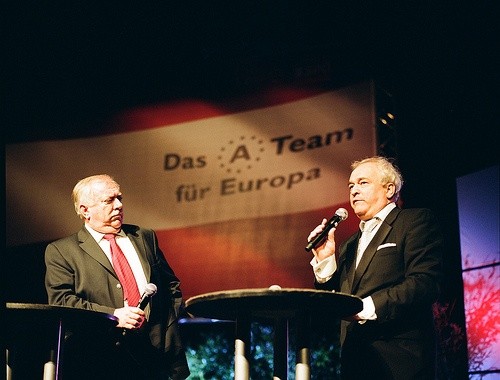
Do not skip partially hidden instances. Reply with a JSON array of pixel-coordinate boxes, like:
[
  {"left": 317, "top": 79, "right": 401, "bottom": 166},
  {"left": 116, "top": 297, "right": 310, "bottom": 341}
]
[
  {"left": 304, "top": 208, "right": 348, "bottom": 252},
  {"left": 122, "top": 283, "right": 157, "bottom": 336}
]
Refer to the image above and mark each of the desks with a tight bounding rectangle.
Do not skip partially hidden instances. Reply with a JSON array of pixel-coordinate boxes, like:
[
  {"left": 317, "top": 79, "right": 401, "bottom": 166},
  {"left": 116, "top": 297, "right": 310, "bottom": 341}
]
[
  {"left": 0, "top": 301, "right": 121, "bottom": 380},
  {"left": 183, "top": 288, "right": 364, "bottom": 380}
]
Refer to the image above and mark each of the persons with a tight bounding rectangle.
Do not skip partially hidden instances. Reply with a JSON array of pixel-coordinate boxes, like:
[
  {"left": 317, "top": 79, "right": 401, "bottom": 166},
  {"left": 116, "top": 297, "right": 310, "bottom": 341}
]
[
  {"left": 44, "top": 174, "right": 185, "bottom": 380},
  {"left": 307, "top": 156, "right": 449, "bottom": 380}
]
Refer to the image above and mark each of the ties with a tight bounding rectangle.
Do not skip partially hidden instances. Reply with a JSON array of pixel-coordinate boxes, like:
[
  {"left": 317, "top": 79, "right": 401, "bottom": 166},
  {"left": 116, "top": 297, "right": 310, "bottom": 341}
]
[{"left": 103, "top": 233, "right": 146, "bottom": 330}]
